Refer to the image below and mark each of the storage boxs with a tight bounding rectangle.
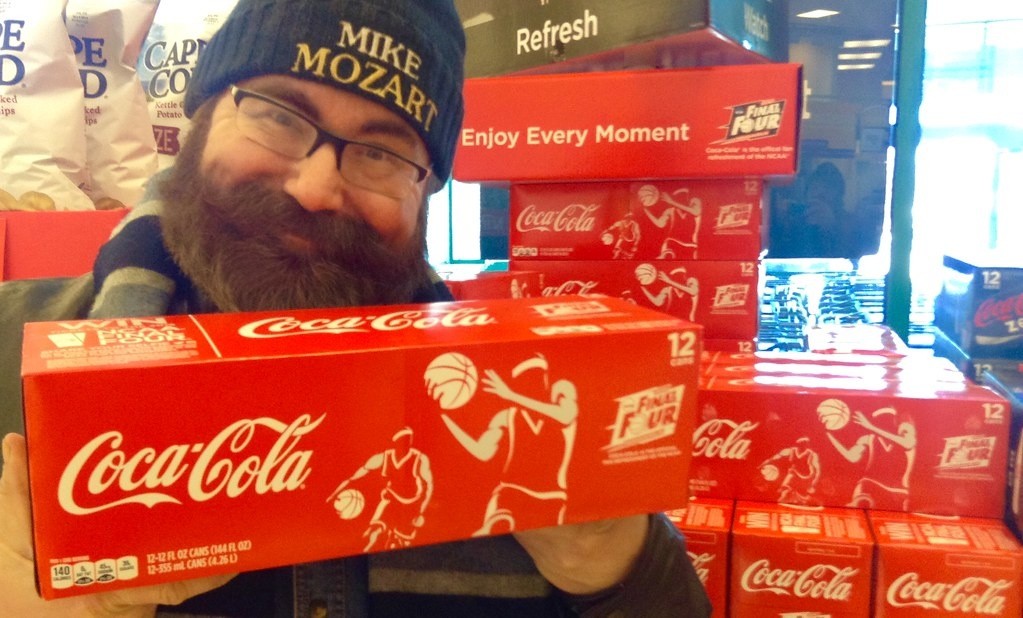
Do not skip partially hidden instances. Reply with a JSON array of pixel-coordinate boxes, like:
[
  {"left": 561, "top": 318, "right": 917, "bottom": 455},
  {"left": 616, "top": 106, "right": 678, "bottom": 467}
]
[
  {"left": 666, "top": 250, "right": 1023, "bottom": 618},
  {"left": 452, "top": 61, "right": 804, "bottom": 189},
  {"left": 20, "top": 294, "right": 702, "bottom": 601},
  {"left": 509, "top": 180, "right": 771, "bottom": 263},
  {"left": 444, "top": 259, "right": 765, "bottom": 341},
  {"left": 450, "top": 1, "right": 787, "bottom": 80}
]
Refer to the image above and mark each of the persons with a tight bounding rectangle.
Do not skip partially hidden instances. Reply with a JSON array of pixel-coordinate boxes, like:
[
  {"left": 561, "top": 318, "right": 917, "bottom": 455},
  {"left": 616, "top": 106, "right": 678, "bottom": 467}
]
[
  {"left": 776, "top": 162, "right": 876, "bottom": 257},
  {"left": 0, "top": 0, "right": 712, "bottom": 618}
]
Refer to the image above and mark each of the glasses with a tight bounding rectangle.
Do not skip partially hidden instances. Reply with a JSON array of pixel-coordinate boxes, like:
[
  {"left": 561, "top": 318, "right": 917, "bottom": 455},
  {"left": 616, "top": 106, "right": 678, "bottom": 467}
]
[{"left": 227, "top": 82, "right": 436, "bottom": 199}]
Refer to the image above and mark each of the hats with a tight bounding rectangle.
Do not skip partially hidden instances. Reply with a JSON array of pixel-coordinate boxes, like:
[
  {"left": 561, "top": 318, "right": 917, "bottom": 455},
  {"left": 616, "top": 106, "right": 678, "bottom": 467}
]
[{"left": 185, "top": 0, "right": 467, "bottom": 195}]
